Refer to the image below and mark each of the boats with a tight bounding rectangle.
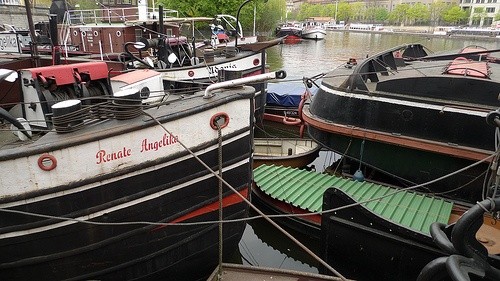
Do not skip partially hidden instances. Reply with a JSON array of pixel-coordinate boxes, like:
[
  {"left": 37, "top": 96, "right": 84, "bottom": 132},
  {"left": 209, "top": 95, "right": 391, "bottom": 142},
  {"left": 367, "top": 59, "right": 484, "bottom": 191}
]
[{"left": 0, "top": 0, "right": 500, "bottom": 281}]
